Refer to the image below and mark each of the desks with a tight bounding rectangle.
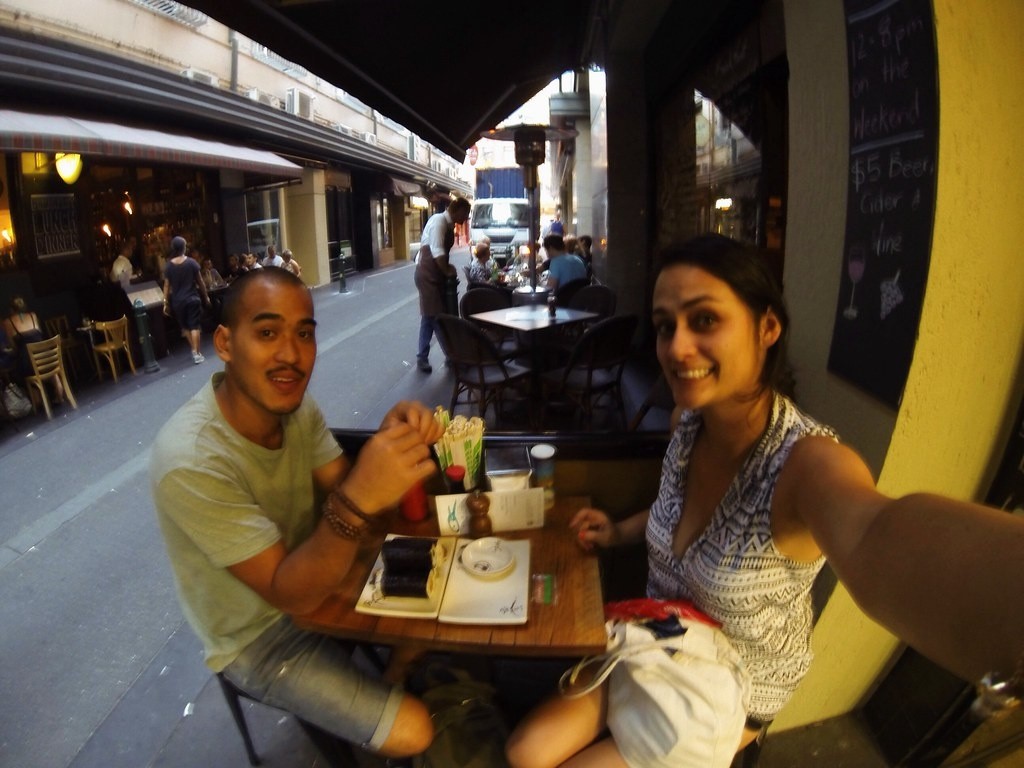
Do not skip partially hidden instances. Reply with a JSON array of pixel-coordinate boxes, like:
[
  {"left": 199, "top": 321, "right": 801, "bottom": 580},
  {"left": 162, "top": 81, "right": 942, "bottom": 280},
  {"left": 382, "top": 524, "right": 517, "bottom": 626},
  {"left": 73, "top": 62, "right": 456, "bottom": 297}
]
[
  {"left": 468, "top": 304, "right": 600, "bottom": 361},
  {"left": 294, "top": 492, "right": 607, "bottom": 656},
  {"left": 64, "top": 322, "right": 112, "bottom": 384}
]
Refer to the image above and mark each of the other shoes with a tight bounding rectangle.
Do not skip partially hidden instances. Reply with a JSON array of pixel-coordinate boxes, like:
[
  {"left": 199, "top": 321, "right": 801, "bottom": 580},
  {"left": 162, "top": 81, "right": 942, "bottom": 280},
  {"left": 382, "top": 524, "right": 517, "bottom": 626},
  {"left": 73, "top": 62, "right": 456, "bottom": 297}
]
[
  {"left": 193, "top": 353, "right": 205, "bottom": 364},
  {"left": 415, "top": 356, "right": 433, "bottom": 371}
]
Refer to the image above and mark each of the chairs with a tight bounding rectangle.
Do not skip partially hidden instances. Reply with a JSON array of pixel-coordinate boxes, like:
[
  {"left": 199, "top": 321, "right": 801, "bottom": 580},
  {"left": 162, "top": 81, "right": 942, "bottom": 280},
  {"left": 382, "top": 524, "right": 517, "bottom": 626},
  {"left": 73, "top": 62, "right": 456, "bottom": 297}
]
[
  {"left": 436, "top": 265, "right": 640, "bottom": 434},
  {"left": 22, "top": 313, "right": 136, "bottom": 422}
]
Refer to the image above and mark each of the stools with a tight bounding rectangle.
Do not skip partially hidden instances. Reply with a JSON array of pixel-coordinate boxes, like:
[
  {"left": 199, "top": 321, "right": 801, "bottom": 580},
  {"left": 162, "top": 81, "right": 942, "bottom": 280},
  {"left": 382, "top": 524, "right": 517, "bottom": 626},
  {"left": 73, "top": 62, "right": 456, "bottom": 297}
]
[{"left": 217, "top": 637, "right": 388, "bottom": 768}]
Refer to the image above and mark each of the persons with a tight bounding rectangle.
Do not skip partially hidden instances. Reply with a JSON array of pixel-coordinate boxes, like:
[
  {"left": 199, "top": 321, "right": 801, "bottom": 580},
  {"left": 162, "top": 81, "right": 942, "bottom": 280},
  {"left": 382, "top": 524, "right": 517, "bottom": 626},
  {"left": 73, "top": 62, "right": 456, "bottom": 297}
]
[
  {"left": 513, "top": 232, "right": 595, "bottom": 301},
  {"left": 542, "top": 213, "right": 564, "bottom": 244},
  {"left": 3, "top": 295, "right": 64, "bottom": 408},
  {"left": 505, "top": 232, "right": 1024, "bottom": 768},
  {"left": 78, "top": 274, "right": 140, "bottom": 374},
  {"left": 192, "top": 245, "right": 301, "bottom": 290},
  {"left": 162, "top": 236, "right": 212, "bottom": 364},
  {"left": 150, "top": 266, "right": 445, "bottom": 758},
  {"left": 110, "top": 232, "right": 144, "bottom": 288},
  {"left": 470, "top": 236, "right": 499, "bottom": 288},
  {"left": 414, "top": 197, "right": 472, "bottom": 372}
]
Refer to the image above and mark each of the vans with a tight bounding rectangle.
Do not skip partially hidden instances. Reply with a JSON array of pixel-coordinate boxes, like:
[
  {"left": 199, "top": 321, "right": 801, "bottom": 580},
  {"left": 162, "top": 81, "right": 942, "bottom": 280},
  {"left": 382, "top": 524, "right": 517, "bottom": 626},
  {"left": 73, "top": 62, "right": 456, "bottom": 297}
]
[{"left": 467, "top": 197, "right": 530, "bottom": 268}]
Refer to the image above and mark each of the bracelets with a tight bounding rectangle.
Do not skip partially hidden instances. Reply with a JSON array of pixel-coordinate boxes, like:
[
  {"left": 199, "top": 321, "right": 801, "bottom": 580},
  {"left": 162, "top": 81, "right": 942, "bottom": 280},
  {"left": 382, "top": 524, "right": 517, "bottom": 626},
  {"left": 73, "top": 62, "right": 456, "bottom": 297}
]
[{"left": 320, "top": 486, "right": 377, "bottom": 541}]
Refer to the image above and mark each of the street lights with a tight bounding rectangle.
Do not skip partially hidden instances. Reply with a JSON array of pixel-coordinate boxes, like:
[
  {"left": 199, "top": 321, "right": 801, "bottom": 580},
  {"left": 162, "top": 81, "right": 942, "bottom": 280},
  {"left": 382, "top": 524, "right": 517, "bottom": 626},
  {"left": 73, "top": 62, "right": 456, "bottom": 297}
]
[{"left": 482, "top": 122, "right": 581, "bottom": 292}]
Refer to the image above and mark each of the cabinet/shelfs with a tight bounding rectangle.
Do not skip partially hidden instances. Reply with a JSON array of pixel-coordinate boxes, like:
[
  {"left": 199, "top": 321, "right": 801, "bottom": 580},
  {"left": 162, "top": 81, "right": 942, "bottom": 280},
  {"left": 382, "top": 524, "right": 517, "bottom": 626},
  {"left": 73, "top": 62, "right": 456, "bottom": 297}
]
[{"left": 84, "top": 166, "right": 227, "bottom": 281}]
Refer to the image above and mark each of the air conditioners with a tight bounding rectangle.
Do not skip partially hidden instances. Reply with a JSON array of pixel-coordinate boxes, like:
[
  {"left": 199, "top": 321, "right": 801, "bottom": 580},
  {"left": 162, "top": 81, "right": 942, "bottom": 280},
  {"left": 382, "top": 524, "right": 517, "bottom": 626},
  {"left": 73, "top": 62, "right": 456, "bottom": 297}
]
[
  {"left": 432, "top": 161, "right": 441, "bottom": 173},
  {"left": 445, "top": 167, "right": 457, "bottom": 180},
  {"left": 286, "top": 87, "right": 315, "bottom": 122},
  {"left": 359, "top": 132, "right": 376, "bottom": 146},
  {"left": 332, "top": 124, "right": 352, "bottom": 136},
  {"left": 245, "top": 88, "right": 280, "bottom": 109},
  {"left": 180, "top": 68, "right": 220, "bottom": 88}
]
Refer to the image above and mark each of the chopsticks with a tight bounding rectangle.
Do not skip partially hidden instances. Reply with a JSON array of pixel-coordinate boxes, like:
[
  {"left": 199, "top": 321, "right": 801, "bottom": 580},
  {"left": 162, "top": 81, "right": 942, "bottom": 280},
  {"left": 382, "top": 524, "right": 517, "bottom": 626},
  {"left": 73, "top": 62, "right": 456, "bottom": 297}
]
[{"left": 432, "top": 405, "right": 484, "bottom": 487}]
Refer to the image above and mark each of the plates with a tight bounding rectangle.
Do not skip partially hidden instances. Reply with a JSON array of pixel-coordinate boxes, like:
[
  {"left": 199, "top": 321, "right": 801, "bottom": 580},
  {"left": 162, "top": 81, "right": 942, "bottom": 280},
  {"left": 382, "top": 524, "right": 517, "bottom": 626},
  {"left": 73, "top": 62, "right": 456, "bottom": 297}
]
[
  {"left": 438, "top": 537, "right": 530, "bottom": 624},
  {"left": 462, "top": 538, "right": 516, "bottom": 576},
  {"left": 355, "top": 531, "right": 457, "bottom": 618}
]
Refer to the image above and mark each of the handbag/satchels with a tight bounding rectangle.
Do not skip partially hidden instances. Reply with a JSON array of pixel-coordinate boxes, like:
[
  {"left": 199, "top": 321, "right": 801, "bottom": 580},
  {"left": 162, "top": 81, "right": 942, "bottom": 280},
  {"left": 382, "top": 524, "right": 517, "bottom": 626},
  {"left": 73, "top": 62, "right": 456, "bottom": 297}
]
[{"left": 558, "top": 598, "right": 753, "bottom": 768}]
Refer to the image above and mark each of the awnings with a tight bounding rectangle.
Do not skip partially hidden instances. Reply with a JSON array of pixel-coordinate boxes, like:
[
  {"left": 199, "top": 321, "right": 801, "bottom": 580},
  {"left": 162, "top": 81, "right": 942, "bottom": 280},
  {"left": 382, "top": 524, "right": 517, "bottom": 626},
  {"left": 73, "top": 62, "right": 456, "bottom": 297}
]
[
  {"left": 0, "top": 109, "right": 303, "bottom": 199},
  {"left": 174, "top": 0, "right": 604, "bottom": 164},
  {"left": 427, "top": 186, "right": 458, "bottom": 204},
  {"left": 271, "top": 151, "right": 352, "bottom": 192},
  {"left": 380, "top": 172, "right": 422, "bottom": 197}
]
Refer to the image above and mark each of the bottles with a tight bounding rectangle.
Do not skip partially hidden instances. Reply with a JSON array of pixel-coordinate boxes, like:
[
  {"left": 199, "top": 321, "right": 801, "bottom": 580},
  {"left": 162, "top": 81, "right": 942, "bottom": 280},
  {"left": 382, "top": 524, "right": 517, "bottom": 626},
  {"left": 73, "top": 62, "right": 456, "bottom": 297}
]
[
  {"left": 548, "top": 292, "right": 557, "bottom": 317},
  {"left": 532, "top": 443, "right": 556, "bottom": 494},
  {"left": 401, "top": 480, "right": 428, "bottom": 521},
  {"left": 446, "top": 465, "right": 467, "bottom": 495}
]
